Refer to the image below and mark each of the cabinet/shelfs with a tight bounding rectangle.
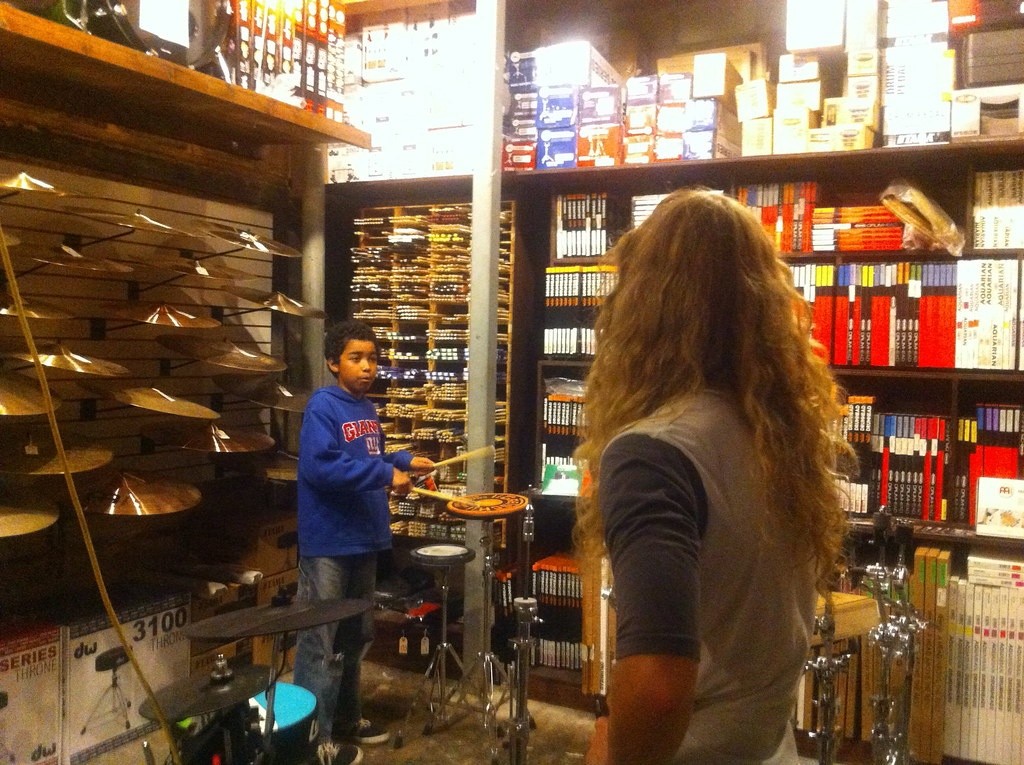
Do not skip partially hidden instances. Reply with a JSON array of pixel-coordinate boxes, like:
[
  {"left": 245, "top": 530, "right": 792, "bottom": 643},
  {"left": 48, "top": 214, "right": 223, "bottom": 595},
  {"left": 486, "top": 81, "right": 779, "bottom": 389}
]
[
  {"left": 514, "top": 138, "right": 1024, "bottom": 765},
  {"left": 324, "top": 174, "right": 513, "bottom": 682}
]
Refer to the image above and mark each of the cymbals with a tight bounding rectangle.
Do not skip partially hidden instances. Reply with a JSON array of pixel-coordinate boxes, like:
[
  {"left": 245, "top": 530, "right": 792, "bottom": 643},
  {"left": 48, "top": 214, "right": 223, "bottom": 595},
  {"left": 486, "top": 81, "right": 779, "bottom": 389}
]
[
  {"left": 61, "top": 205, "right": 209, "bottom": 238},
  {"left": 0, "top": 424, "right": 114, "bottom": 475},
  {"left": 212, "top": 373, "right": 314, "bottom": 413},
  {"left": 0, "top": 233, "right": 20, "bottom": 249},
  {"left": 0, "top": 172, "right": 84, "bottom": 196},
  {"left": 0, "top": 343, "right": 133, "bottom": 377},
  {"left": 76, "top": 382, "right": 221, "bottom": 420},
  {"left": 31, "top": 254, "right": 135, "bottom": 273},
  {"left": 138, "top": 664, "right": 277, "bottom": 721},
  {"left": 192, "top": 219, "right": 302, "bottom": 258},
  {"left": 207, "top": 448, "right": 300, "bottom": 481},
  {"left": 154, "top": 334, "right": 288, "bottom": 372},
  {"left": 44, "top": 469, "right": 203, "bottom": 517},
  {"left": 182, "top": 598, "right": 374, "bottom": 639},
  {"left": 220, "top": 285, "right": 329, "bottom": 319},
  {"left": 0, "top": 370, "right": 63, "bottom": 417},
  {"left": 0, "top": 483, "right": 61, "bottom": 539},
  {"left": 140, "top": 423, "right": 276, "bottom": 453},
  {"left": 0, "top": 295, "right": 79, "bottom": 319},
  {"left": 127, "top": 255, "right": 257, "bottom": 281},
  {"left": 99, "top": 302, "right": 222, "bottom": 329}
]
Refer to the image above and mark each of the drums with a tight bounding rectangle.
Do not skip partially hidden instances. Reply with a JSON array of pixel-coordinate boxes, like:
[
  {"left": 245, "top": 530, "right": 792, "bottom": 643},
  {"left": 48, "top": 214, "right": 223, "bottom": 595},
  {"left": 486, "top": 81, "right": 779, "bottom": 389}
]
[
  {"left": 444, "top": 493, "right": 530, "bottom": 521},
  {"left": 409, "top": 543, "right": 477, "bottom": 567},
  {"left": 174, "top": 680, "right": 320, "bottom": 765}
]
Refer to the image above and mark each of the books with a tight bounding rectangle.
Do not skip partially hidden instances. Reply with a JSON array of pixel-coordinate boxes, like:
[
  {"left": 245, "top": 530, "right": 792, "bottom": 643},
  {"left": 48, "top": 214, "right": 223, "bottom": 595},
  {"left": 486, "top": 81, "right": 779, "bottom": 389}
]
[{"left": 533, "top": 191, "right": 1024, "bottom": 764}]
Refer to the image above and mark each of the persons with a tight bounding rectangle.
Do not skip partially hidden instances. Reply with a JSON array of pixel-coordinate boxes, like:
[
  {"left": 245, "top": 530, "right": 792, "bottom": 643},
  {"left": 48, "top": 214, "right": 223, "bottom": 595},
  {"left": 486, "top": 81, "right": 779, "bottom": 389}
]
[
  {"left": 295, "top": 321, "right": 436, "bottom": 765},
  {"left": 584, "top": 190, "right": 852, "bottom": 765}
]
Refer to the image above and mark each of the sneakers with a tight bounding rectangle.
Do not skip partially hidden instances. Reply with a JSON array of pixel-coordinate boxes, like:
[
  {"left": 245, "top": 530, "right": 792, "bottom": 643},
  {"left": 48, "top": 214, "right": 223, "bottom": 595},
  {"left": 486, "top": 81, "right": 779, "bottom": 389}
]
[
  {"left": 347, "top": 718, "right": 389, "bottom": 743},
  {"left": 314, "top": 742, "right": 363, "bottom": 765}
]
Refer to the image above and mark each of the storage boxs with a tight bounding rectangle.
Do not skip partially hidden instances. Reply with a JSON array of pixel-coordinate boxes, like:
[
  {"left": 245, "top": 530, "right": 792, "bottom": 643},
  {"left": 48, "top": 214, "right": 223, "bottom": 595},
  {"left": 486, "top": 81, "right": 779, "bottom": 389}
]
[
  {"left": 536, "top": 86, "right": 581, "bottom": 130},
  {"left": 579, "top": 85, "right": 624, "bottom": 126},
  {"left": 577, "top": 126, "right": 623, "bottom": 167},
  {"left": 508, "top": 51, "right": 540, "bottom": 93},
  {"left": 693, "top": 52, "right": 744, "bottom": 115},
  {"left": 390, "top": 544, "right": 465, "bottom": 624},
  {"left": 0, "top": 508, "right": 299, "bottom": 765},
  {"left": 622, "top": 136, "right": 656, "bottom": 165},
  {"left": 660, "top": 72, "right": 693, "bottom": 103},
  {"left": 625, "top": 73, "right": 660, "bottom": 106},
  {"left": 502, "top": 138, "right": 536, "bottom": 173},
  {"left": 537, "top": 130, "right": 578, "bottom": 169},
  {"left": 684, "top": 99, "right": 742, "bottom": 146},
  {"left": 734, "top": 0, "right": 1024, "bottom": 156},
  {"left": 654, "top": 133, "right": 683, "bottom": 161},
  {"left": 682, "top": 131, "right": 741, "bottom": 160},
  {"left": 509, "top": 93, "right": 537, "bottom": 119},
  {"left": 624, "top": 105, "right": 657, "bottom": 137},
  {"left": 658, "top": 103, "right": 684, "bottom": 135},
  {"left": 538, "top": 40, "right": 622, "bottom": 88}
]
